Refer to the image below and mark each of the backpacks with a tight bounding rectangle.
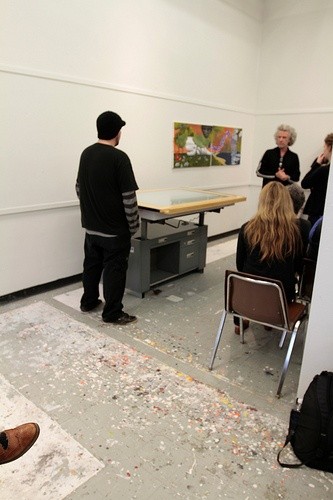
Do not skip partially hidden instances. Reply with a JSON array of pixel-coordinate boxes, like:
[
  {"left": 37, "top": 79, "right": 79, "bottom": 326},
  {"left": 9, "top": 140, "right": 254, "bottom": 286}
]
[{"left": 287, "top": 371, "right": 333, "bottom": 472}]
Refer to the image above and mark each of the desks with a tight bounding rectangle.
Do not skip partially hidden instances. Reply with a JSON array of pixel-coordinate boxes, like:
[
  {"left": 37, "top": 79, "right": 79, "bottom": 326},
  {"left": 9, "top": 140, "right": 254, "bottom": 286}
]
[{"left": 125, "top": 184, "right": 249, "bottom": 299}]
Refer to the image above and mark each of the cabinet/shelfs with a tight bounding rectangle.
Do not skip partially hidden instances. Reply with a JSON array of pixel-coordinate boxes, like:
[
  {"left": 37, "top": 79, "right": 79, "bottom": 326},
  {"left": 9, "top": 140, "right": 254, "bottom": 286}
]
[{"left": 125, "top": 218, "right": 209, "bottom": 300}]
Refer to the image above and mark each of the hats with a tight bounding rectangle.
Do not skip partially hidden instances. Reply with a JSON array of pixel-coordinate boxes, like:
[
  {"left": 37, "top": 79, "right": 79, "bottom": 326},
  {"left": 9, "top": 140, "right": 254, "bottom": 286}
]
[{"left": 97, "top": 111, "right": 126, "bottom": 140}]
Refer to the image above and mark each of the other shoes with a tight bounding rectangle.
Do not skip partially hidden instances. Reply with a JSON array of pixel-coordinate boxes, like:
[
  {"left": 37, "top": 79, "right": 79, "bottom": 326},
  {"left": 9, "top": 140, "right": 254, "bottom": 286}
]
[{"left": 236, "top": 319, "right": 250, "bottom": 335}]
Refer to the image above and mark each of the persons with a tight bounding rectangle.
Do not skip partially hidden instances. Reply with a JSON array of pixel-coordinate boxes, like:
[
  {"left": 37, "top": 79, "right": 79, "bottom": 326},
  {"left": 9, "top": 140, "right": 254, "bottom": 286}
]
[
  {"left": 0, "top": 422, "right": 40, "bottom": 466},
  {"left": 75, "top": 111, "right": 137, "bottom": 325},
  {"left": 233, "top": 181, "right": 303, "bottom": 335},
  {"left": 286, "top": 183, "right": 311, "bottom": 274},
  {"left": 256, "top": 124, "right": 301, "bottom": 188},
  {"left": 297, "top": 133, "right": 333, "bottom": 299}
]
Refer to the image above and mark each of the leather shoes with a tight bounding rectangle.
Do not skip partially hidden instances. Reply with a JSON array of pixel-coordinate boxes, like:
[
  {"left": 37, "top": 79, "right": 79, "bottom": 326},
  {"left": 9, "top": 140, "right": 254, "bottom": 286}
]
[
  {"left": 0, "top": 423, "right": 40, "bottom": 465},
  {"left": 81, "top": 298, "right": 102, "bottom": 312},
  {"left": 115, "top": 311, "right": 136, "bottom": 324}
]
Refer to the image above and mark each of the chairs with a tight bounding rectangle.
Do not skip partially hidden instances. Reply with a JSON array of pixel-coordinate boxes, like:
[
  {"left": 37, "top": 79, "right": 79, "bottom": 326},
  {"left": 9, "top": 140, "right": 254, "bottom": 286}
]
[{"left": 208, "top": 269, "right": 309, "bottom": 399}]
